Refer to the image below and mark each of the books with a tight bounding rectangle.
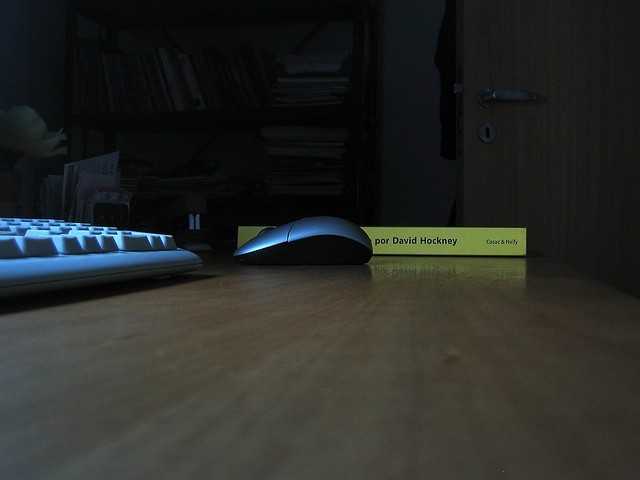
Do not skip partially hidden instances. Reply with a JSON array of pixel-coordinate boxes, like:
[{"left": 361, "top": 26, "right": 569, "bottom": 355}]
[{"left": 231, "top": 223, "right": 529, "bottom": 258}]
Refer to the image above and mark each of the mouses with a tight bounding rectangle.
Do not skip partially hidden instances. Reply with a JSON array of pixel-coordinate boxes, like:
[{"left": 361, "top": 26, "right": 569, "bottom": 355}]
[{"left": 233, "top": 215, "right": 373, "bottom": 267}]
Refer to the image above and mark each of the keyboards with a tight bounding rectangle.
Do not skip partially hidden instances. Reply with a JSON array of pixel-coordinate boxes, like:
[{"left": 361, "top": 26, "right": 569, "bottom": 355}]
[{"left": 0, "top": 219, "right": 205, "bottom": 296}]
